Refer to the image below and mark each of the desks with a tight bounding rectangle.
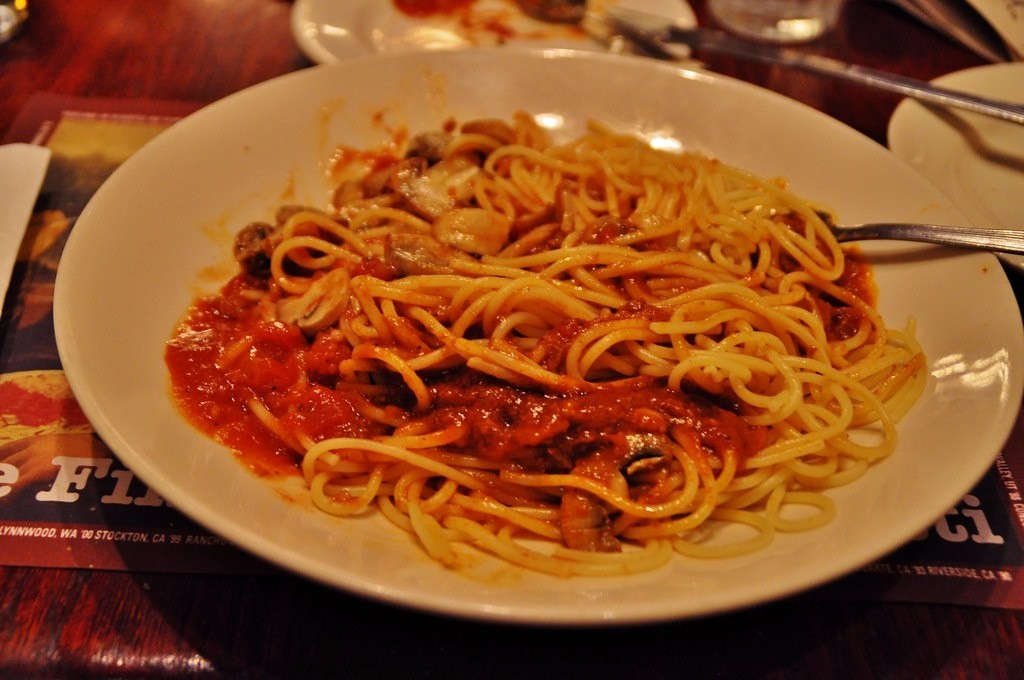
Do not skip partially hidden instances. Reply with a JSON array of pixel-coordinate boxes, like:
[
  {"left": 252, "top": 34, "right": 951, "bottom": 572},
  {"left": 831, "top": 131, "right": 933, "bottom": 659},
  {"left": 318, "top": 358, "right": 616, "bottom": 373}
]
[{"left": 0, "top": 0, "right": 1024, "bottom": 680}]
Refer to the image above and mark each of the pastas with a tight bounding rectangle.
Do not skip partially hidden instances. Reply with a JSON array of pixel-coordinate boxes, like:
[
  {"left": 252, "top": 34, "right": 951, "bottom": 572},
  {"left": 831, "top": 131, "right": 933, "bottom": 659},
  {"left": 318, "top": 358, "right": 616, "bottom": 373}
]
[{"left": 219, "top": 107, "right": 924, "bottom": 578}]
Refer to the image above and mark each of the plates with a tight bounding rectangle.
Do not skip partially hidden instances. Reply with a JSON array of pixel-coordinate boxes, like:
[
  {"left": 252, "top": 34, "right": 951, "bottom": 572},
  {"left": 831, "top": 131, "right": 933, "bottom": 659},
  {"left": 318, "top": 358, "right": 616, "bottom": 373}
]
[
  {"left": 53, "top": 45, "right": 1024, "bottom": 623},
  {"left": 887, "top": 62, "right": 1024, "bottom": 265},
  {"left": 291, "top": 0, "right": 698, "bottom": 64}
]
